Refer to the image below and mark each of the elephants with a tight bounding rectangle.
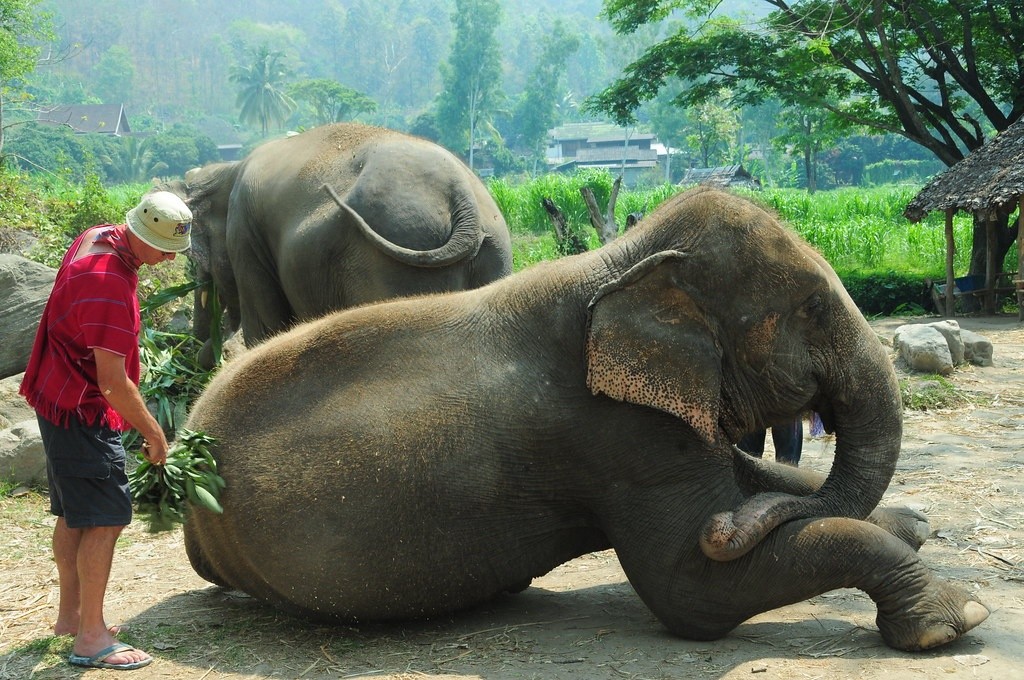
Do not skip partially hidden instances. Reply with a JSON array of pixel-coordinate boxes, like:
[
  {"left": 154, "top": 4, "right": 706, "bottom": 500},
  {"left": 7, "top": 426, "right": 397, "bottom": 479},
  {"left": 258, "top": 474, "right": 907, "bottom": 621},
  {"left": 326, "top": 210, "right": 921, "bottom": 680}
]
[
  {"left": 163, "top": 183, "right": 992, "bottom": 654},
  {"left": 139, "top": 120, "right": 514, "bottom": 379}
]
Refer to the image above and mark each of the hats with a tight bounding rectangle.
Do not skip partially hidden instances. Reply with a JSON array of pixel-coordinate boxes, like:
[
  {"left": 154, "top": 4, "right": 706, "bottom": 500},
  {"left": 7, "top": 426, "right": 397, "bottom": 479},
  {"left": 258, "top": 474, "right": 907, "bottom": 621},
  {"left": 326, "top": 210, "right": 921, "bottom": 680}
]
[{"left": 126, "top": 191, "right": 194, "bottom": 253}]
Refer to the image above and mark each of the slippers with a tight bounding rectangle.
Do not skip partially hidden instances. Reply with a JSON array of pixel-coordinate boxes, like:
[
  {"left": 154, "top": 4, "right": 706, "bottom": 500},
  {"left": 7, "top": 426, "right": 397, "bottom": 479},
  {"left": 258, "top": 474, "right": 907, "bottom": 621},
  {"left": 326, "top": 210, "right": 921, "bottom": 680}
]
[
  {"left": 107, "top": 623, "right": 130, "bottom": 638},
  {"left": 69, "top": 641, "right": 153, "bottom": 670}
]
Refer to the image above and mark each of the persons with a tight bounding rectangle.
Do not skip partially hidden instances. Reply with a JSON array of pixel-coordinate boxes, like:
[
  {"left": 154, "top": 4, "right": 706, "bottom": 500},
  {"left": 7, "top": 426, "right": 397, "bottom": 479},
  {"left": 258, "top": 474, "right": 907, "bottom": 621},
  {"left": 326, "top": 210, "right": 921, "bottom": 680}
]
[{"left": 18, "top": 191, "right": 193, "bottom": 669}]
